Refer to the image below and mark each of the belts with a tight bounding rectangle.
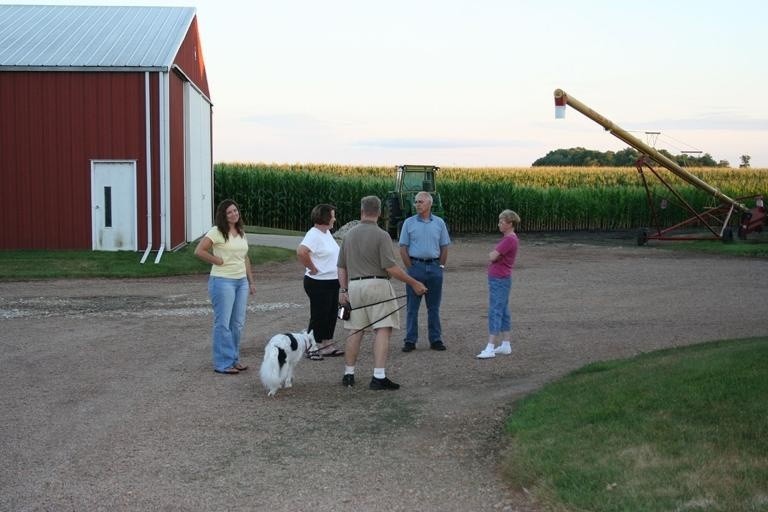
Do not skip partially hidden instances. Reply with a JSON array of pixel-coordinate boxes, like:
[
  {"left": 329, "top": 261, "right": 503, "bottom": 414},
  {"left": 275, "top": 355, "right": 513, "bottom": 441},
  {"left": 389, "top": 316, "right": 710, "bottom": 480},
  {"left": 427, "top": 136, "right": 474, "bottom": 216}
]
[
  {"left": 412, "top": 258, "right": 439, "bottom": 263},
  {"left": 352, "top": 276, "right": 386, "bottom": 280}
]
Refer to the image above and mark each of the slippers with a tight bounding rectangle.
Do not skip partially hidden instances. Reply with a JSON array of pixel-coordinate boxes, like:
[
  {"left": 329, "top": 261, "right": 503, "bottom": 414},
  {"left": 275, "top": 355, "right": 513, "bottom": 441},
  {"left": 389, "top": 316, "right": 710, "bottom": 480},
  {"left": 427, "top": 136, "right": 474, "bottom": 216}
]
[
  {"left": 321, "top": 349, "right": 344, "bottom": 357},
  {"left": 214, "top": 364, "right": 248, "bottom": 373}
]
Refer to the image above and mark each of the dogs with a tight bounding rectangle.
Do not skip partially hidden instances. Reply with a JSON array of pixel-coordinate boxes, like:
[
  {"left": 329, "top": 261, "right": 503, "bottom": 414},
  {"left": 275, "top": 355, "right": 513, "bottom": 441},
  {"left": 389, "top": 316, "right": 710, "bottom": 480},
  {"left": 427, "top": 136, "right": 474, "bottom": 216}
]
[{"left": 258, "top": 327, "right": 318, "bottom": 396}]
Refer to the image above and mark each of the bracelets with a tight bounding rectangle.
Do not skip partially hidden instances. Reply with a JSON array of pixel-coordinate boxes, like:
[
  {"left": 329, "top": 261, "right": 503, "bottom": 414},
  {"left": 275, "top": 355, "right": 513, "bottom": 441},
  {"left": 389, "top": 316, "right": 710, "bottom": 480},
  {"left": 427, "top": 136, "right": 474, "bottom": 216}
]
[
  {"left": 337, "top": 286, "right": 348, "bottom": 294},
  {"left": 440, "top": 264, "right": 446, "bottom": 271}
]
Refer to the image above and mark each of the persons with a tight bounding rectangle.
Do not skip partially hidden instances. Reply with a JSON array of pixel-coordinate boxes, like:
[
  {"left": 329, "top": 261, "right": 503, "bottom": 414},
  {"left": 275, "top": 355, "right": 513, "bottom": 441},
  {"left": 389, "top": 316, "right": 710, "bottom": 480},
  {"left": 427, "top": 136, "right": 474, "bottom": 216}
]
[
  {"left": 194, "top": 198, "right": 258, "bottom": 375},
  {"left": 336, "top": 193, "right": 430, "bottom": 391},
  {"left": 397, "top": 190, "right": 452, "bottom": 353},
  {"left": 296, "top": 204, "right": 347, "bottom": 358},
  {"left": 474, "top": 210, "right": 523, "bottom": 362}
]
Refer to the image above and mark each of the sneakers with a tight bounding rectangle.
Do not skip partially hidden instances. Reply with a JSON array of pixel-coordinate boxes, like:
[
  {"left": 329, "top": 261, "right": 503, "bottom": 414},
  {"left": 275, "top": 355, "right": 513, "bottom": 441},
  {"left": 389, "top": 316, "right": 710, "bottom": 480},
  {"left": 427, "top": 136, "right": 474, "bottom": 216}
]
[
  {"left": 370, "top": 375, "right": 399, "bottom": 390},
  {"left": 402, "top": 342, "right": 415, "bottom": 352},
  {"left": 495, "top": 346, "right": 511, "bottom": 355},
  {"left": 432, "top": 341, "right": 446, "bottom": 350},
  {"left": 475, "top": 349, "right": 496, "bottom": 358},
  {"left": 343, "top": 374, "right": 355, "bottom": 386}
]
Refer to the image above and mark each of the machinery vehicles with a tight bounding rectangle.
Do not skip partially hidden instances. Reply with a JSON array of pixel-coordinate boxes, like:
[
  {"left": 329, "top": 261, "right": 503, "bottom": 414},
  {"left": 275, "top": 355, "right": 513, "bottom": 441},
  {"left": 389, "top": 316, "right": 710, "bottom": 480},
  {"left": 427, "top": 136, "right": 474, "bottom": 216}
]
[
  {"left": 547, "top": 88, "right": 767, "bottom": 246},
  {"left": 379, "top": 163, "right": 451, "bottom": 240}
]
